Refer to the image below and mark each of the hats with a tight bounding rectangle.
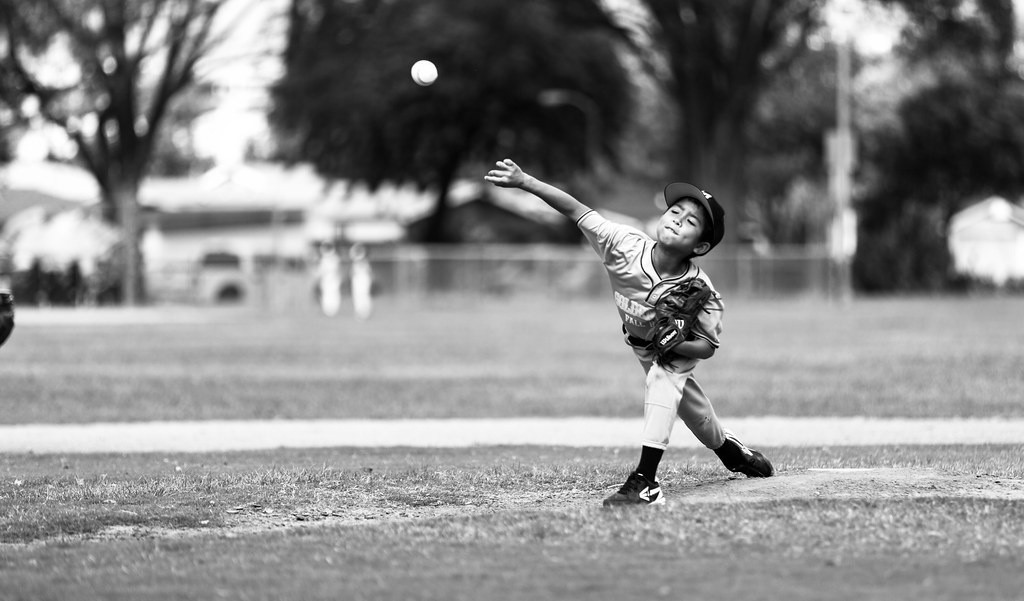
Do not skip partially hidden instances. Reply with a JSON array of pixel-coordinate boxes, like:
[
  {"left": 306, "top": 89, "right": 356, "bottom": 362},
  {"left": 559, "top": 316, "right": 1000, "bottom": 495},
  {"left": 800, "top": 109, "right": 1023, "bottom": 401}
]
[{"left": 664, "top": 181, "right": 725, "bottom": 247}]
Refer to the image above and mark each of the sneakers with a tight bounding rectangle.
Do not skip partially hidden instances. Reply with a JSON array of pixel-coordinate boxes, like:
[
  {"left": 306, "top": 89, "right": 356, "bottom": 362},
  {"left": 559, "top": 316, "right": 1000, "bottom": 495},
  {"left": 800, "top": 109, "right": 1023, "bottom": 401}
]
[
  {"left": 714, "top": 427, "right": 774, "bottom": 478},
  {"left": 603, "top": 471, "right": 666, "bottom": 505}
]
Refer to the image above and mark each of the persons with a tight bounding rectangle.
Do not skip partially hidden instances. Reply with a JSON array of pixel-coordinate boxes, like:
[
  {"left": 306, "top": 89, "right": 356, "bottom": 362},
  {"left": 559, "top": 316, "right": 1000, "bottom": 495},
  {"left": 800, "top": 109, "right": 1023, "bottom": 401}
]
[{"left": 484, "top": 158, "right": 773, "bottom": 507}]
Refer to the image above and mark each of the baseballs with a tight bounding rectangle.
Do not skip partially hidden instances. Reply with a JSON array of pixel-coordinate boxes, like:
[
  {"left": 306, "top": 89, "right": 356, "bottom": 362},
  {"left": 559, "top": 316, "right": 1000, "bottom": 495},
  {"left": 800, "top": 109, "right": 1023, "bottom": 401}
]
[{"left": 410, "top": 59, "right": 439, "bottom": 86}]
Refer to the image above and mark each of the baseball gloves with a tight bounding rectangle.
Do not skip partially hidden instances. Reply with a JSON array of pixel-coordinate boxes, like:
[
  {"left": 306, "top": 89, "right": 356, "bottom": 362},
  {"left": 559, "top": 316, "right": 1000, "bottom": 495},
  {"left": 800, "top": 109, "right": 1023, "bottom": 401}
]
[{"left": 645, "top": 275, "right": 716, "bottom": 375}]
